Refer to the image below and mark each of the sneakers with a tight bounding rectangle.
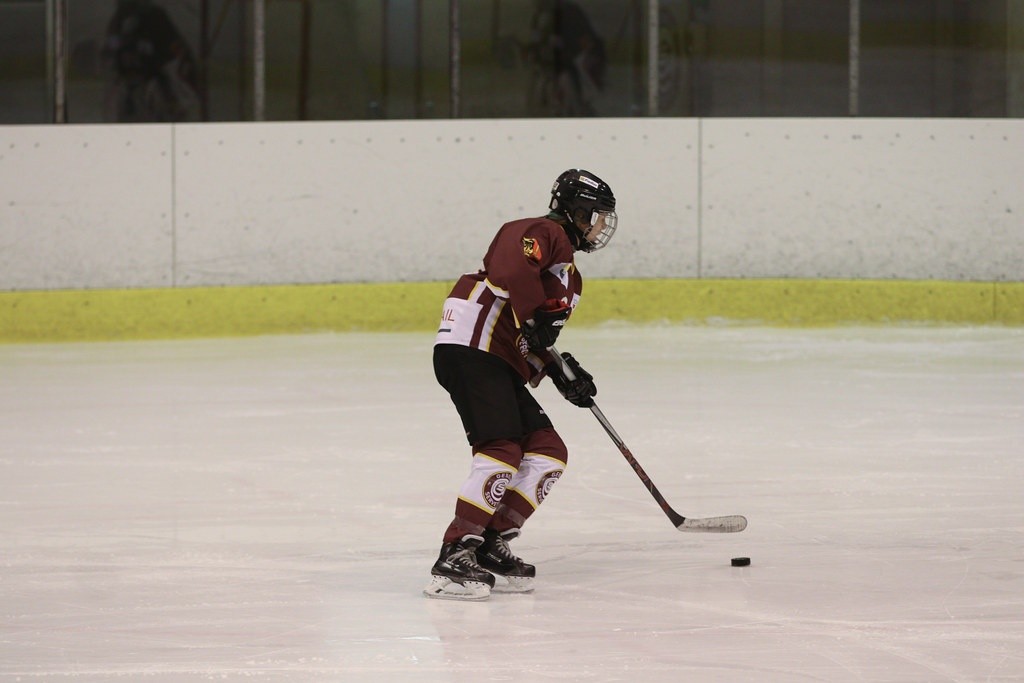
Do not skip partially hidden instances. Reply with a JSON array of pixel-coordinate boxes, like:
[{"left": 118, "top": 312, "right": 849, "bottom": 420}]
[
  {"left": 423, "top": 533, "right": 497, "bottom": 602},
  {"left": 474, "top": 527, "right": 537, "bottom": 593}
]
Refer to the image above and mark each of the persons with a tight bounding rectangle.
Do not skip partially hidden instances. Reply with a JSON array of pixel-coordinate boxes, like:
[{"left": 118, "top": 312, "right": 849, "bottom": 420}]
[{"left": 432, "top": 168, "right": 617, "bottom": 588}]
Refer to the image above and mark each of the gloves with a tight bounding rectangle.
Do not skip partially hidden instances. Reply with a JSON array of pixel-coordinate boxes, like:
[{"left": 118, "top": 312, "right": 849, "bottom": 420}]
[
  {"left": 547, "top": 351, "right": 597, "bottom": 409},
  {"left": 522, "top": 308, "right": 573, "bottom": 352}
]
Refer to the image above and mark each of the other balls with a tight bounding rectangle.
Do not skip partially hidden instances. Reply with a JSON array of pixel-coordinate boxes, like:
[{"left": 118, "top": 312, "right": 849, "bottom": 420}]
[{"left": 730, "top": 557, "right": 751, "bottom": 567}]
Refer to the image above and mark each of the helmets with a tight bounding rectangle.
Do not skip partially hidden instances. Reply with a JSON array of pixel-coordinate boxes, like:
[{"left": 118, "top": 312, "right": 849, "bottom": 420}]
[{"left": 547, "top": 168, "right": 619, "bottom": 255}]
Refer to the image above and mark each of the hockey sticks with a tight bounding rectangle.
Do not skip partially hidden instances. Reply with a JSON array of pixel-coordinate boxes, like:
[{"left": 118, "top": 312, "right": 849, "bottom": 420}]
[{"left": 546, "top": 347, "right": 749, "bottom": 534}]
